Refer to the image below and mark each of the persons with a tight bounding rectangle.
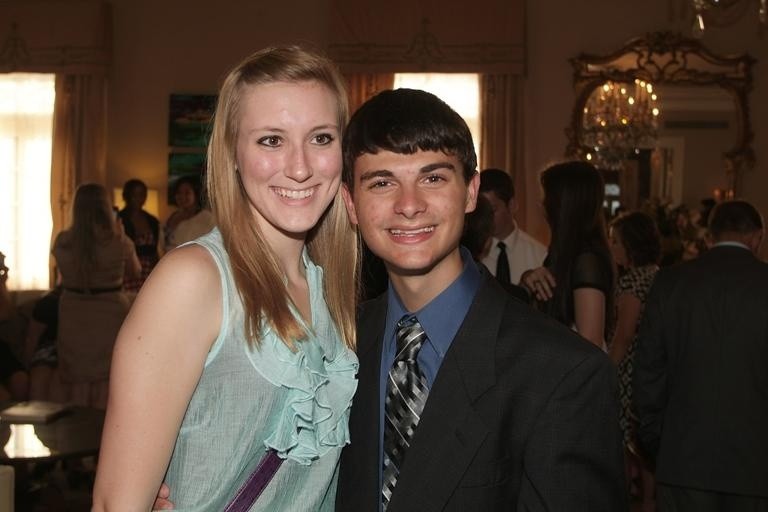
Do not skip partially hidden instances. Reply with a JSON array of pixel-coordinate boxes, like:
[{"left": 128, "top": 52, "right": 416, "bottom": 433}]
[
  {"left": 88, "top": 43, "right": 359, "bottom": 512},
  {"left": 336, "top": 90, "right": 625, "bottom": 512}
]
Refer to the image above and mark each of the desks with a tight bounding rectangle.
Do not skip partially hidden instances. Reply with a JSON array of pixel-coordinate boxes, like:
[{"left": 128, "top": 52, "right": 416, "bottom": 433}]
[{"left": 0, "top": 405, "right": 106, "bottom": 512}]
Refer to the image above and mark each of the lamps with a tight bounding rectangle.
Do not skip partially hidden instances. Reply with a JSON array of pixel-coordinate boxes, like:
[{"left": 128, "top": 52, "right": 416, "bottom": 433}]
[{"left": 572, "top": 74, "right": 661, "bottom": 170}]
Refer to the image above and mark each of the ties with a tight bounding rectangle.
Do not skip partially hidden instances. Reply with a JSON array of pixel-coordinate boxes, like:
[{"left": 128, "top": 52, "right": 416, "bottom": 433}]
[
  {"left": 497, "top": 242, "right": 511, "bottom": 283},
  {"left": 382, "top": 315, "right": 429, "bottom": 511}
]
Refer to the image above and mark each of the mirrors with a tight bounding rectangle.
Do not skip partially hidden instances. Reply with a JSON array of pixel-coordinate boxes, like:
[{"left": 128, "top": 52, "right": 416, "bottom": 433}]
[{"left": 565, "top": 30, "right": 757, "bottom": 267}]
